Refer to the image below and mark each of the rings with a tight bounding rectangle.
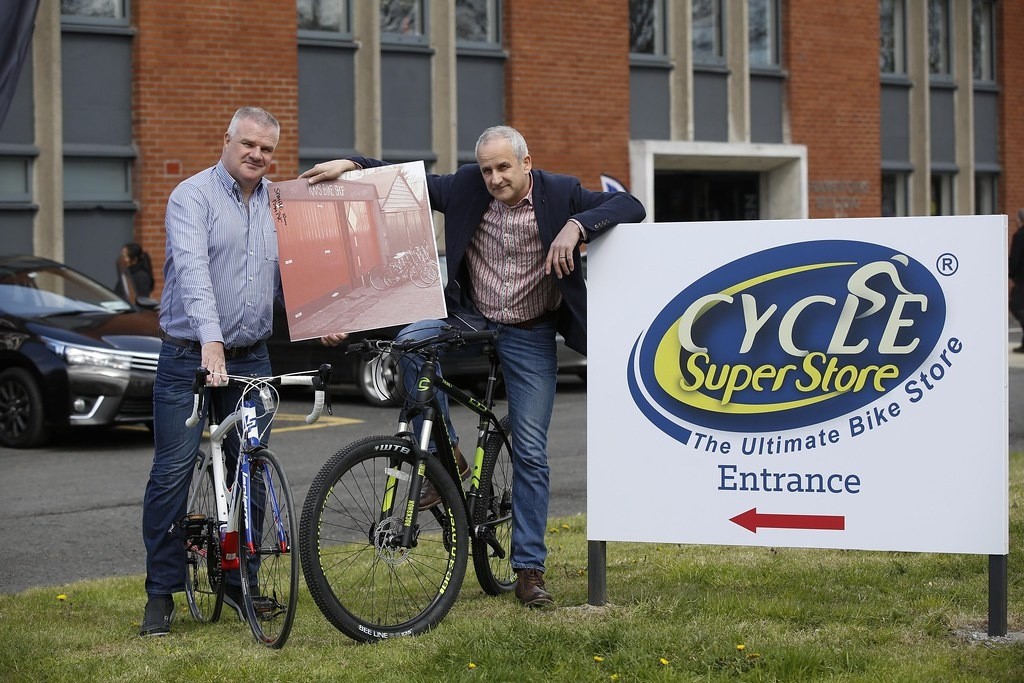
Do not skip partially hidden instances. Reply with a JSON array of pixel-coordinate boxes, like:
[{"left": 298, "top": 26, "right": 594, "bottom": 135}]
[{"left": 559, "top": 256, "right": 565, "bottom": 258}]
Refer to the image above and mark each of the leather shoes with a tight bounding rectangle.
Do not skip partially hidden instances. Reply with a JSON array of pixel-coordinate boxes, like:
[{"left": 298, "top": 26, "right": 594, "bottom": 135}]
[
  {"left": 515, "top": 568, "right": 554, "bottom": 608},
  {"left": 418, "top": 445, "right": 472, "bottom": 511}
]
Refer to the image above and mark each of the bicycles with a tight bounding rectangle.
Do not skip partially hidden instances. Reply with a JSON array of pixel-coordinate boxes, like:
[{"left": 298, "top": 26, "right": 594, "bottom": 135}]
[
  {"left": 179, "top": 364, "right": 333, "bottom": 648},
  {"left": 299, "top": 326, "right": 518, "bottom": 643}
]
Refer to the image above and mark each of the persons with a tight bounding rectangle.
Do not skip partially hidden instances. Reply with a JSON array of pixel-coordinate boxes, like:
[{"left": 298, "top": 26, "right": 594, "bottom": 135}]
[
  {"left": 1009, "top": 209, "right": 1024, "bottom": 353},
  {"left": 114, "top": 243, "right": 154, "bottom": 301},
  {"left": 141, "top": 105, "right": 349, "bottom": 635},
  {"left": 298, "top": 125, "right": 647, "bottom": 608}
]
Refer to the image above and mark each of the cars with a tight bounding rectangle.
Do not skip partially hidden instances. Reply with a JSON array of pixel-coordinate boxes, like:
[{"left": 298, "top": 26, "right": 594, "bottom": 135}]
[
  {"left": 266, "top": 250, "right": 501, "bottom": 408},
  {"left": 555, "top": 251, "right": 587, "bottom": 383},
  {"left": 0, "top": 254, "right": 165, "bottom": 449}
]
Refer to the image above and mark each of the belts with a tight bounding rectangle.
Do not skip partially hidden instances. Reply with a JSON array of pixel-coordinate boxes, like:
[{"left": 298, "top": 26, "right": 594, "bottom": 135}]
[{"left": 163, "top": 333, "right": 263, "bottom": 359}]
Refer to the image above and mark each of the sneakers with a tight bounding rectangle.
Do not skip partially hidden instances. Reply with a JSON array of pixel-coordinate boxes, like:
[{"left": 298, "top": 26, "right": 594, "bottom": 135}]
[
  {"left": 222, "top": 584, "right": 265, "bottom": 623},
  {"left": 140, "top": 595, "right": 175, "bottom": 637}
]
